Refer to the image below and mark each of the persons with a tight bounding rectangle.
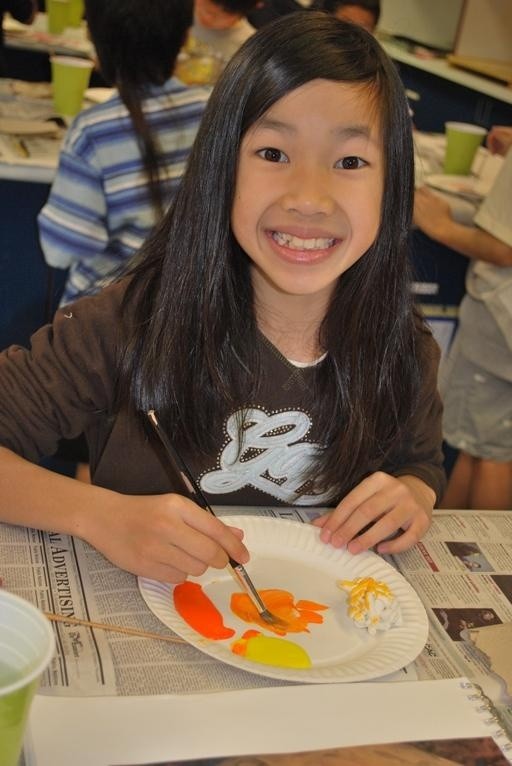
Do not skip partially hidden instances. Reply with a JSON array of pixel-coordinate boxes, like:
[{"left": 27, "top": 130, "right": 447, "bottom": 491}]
[
  {"left": 309, "top": 0, "right": 380, "bottom": 32},
  {"left": 35, "top": 0, "right": 207, "bottom": 313},
  {"left": 407, "top": 120, "right": 510, "bottom": 510},
  {"left": 190, "top": 0, "right": 259, "bottom": 63},
  {"left": 0, "top": 9, "right": 449, "bottom": 587}
]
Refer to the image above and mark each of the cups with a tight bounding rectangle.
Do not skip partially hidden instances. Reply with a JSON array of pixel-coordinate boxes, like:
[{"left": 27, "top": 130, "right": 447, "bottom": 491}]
[
  {"left": 442, "top": 122, "right": 487, "bottom": 175},
  {"left": 0, "top": 589, "right": 55, "bottom": 766},
  {"left": 46, "top": 0, "right": 85, "bottom": 37},
  {"left": 50, "top": 56, "right": 94, "bottom": 119}
]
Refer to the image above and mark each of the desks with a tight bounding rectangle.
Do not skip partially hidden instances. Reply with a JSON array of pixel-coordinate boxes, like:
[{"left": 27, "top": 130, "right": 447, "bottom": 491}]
[
  {"left": 1, "top": 501, "right": 510, "bottom": 766},
  {"left": 0, "top": 9, "right": 496, "bottom": 207}
]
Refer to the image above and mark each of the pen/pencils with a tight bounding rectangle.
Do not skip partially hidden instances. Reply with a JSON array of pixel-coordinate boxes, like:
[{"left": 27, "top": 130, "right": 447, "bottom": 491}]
[{"left": 20, "top": 140, "right": 31, "bottom": 157}]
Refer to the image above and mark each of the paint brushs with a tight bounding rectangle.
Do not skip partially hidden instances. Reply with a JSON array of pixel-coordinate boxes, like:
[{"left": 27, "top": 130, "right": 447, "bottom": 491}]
[{"left": 146, "top": 408, "right": 277, "bottom": 624}]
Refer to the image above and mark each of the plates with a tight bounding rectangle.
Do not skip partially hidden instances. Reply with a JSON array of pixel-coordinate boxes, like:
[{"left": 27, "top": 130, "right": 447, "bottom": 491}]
[{"left": 137, "top": 515, "right": 429, "bottom": 683}]
